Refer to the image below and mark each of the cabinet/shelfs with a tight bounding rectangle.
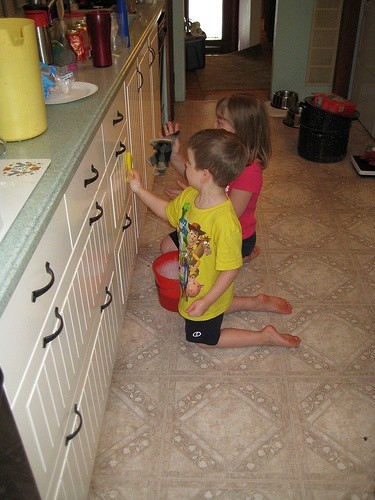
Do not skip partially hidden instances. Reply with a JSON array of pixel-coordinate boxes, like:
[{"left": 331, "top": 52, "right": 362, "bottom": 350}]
[{"left": 0, "top": 3, "right": 170, "bottom": 500}]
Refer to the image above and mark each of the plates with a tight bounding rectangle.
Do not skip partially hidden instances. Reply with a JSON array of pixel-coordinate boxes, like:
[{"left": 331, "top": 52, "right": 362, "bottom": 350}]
[{"left": 45, "top": 82, "right": 98, "bottom": 104}]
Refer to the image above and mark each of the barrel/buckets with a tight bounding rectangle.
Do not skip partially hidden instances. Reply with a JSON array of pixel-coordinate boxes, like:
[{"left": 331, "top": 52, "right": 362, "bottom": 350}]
[
  {"left": 153, "top": 251, "right": 180, "bottom": 312},
  {"left": 298, "top": 97, "right": 360, "bottom": 162}
]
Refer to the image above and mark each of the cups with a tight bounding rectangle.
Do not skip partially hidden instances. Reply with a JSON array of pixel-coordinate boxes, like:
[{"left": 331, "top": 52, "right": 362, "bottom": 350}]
[
  {"left": 87, "top": 11, "right": 112, "bottom": 66},
  {"left": 112, "top": 36, "right": 128, "bottom": 55},
  {"left": 22, "top": 4, "right": 54, "bottom": 65}
]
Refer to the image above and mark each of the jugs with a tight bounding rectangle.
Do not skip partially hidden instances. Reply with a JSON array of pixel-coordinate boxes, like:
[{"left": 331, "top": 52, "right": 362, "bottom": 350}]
[{"left": 0, "top": 17, "right": 48, "bottom": 142}]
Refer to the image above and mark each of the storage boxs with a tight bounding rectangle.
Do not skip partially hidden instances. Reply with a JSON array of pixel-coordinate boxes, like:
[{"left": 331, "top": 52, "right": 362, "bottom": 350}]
[
  {"left": 311, "top": 92, "right": 357, "bottom": 115},
  {"left": 50, "top": 11, "right": 139, "bottom": 49}
]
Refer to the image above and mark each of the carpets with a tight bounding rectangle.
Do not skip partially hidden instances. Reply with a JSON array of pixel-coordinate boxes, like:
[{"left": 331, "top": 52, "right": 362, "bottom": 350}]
[{"left": 195, "top": 44, "right": 272, "bottom": 91}]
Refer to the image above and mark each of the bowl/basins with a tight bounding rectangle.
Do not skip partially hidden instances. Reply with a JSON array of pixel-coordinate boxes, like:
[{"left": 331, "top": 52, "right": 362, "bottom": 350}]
[{"left": 272, "top": 90, "right": 298, "bottom": 109}]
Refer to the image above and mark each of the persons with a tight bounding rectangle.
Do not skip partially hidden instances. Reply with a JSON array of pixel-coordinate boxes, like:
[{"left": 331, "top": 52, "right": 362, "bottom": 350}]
[
  {"left": 158, "top": 93, "right": 271, "bottom": 266},
  {"left": 129, "top": 128, "right": 302, "bottom": 347}
]
